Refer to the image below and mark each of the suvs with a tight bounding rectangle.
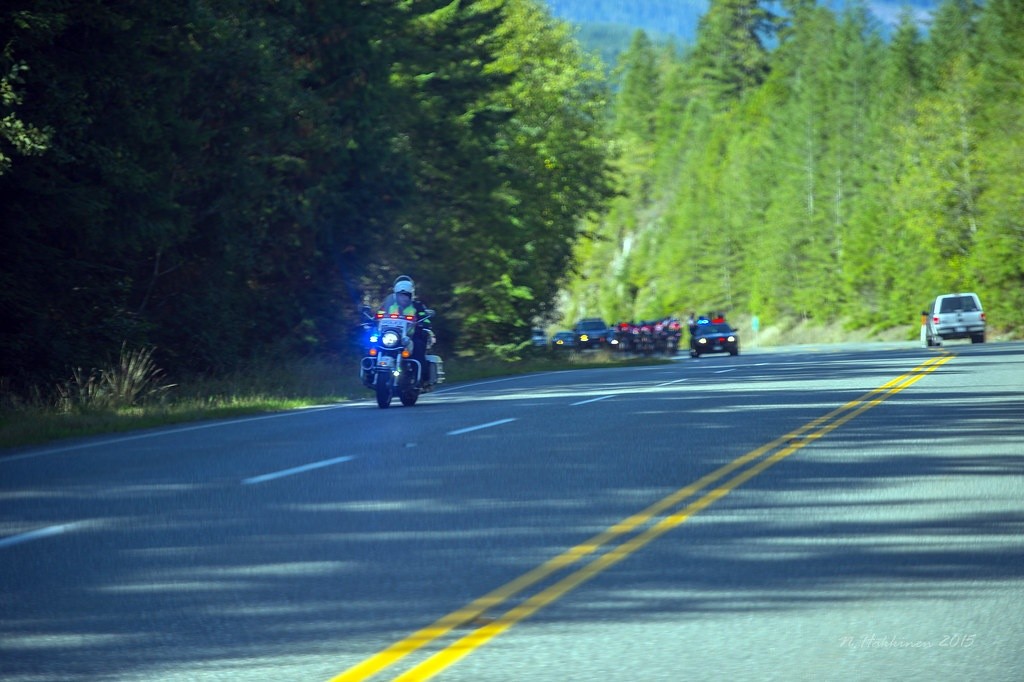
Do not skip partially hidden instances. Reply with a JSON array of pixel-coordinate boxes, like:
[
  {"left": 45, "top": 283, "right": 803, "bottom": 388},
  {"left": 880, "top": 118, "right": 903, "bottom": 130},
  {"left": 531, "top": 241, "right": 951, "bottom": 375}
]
[
  {"left": 531, "top": 329, "right": 547, "bottom": 349},
  {"left": 574, "top": 318, "right": 616, "bottom": 344},
  {"left": 922, "top": 293, "right": 987, "bottom": 347}
]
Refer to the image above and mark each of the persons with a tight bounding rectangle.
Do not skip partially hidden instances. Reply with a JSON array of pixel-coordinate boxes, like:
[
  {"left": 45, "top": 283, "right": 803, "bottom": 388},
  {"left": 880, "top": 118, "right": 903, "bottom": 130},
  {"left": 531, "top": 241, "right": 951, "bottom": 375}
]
[
  {"left": 363, "top": 276, "right": 431, "bottom": 391},
  {"left": 606, "top": 312, "right": 728, "bottom": 330}
]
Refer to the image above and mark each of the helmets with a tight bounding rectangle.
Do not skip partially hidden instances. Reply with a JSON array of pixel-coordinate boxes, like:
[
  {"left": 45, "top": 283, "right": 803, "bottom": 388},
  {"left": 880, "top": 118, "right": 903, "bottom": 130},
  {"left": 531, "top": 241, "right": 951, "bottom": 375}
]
[{"left": 393, "top": 281, "right": 415, "bottom": 301}]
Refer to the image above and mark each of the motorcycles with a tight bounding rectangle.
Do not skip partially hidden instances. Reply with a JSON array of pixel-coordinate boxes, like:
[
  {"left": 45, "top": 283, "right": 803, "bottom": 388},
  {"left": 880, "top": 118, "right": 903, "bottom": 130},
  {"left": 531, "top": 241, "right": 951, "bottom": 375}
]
[
  {"left": 358, "top": 305, "right": 445, "bottom": 410},
  {"left": 609, "top": 317, "right": 681, "bottom": 356}
]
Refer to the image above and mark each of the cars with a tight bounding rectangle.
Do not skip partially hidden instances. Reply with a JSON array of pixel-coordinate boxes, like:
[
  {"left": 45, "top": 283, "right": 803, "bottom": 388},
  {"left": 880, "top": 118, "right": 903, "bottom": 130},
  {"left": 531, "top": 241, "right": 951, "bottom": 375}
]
[
  {"left": 550, "top": 330, "right": 579, "bottom": 350},
  {"left": 689, "top": 315, "right": 741, "bottom": 359}
]
